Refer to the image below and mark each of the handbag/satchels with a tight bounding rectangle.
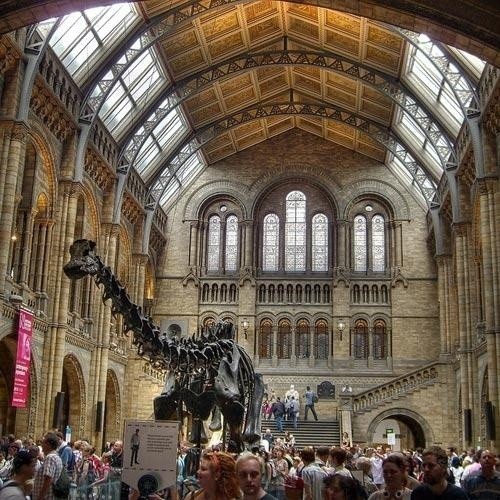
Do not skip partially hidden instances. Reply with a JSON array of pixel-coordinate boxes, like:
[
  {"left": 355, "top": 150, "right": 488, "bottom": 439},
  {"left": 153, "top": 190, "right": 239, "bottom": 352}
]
[
  {"left": 312, "top": 393, "right": 319, "bottom": 403},
  {"left": 86, "top": 461, "right": 97, "bottom": 487},
  {"left": 51, "top": 465, "right": 72, "bottom": 499}
]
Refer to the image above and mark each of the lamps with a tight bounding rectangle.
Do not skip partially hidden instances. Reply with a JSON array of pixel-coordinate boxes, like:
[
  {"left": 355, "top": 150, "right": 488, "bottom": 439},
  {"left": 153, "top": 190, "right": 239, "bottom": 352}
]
[
  {"left": 239, "top": 318, "right": 251, "bottom": 340},
  {"left": 336, "top": 319, "right": 348, "bottom": 340}
]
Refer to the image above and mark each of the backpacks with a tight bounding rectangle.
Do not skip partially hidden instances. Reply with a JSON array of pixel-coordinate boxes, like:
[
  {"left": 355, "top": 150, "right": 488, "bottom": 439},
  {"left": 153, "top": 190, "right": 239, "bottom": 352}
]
[{"left": 67, "top": 453, "right": 75, "bottom": 474}]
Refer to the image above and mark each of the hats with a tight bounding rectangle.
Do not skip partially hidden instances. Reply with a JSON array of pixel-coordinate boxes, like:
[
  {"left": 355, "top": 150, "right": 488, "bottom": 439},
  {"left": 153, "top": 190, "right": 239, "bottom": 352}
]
[{"left": 292, "top": 456, "right": 302, "bottom": 463}]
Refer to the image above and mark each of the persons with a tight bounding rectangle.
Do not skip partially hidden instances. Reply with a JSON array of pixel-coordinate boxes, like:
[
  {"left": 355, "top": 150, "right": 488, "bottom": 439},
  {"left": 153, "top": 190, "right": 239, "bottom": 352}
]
[{"left": 0, "top": 382, "right": 500, "bottom": 500}]
